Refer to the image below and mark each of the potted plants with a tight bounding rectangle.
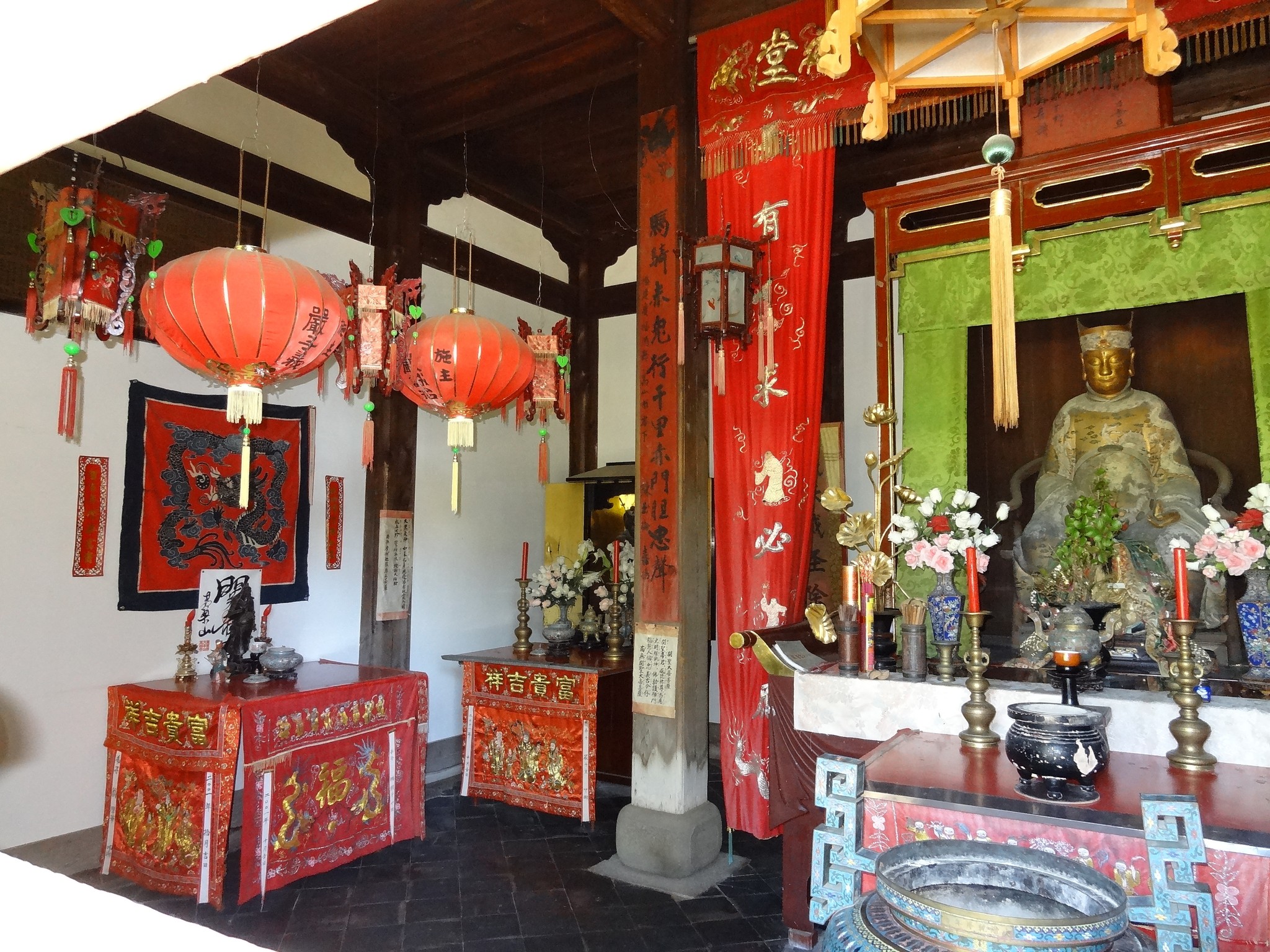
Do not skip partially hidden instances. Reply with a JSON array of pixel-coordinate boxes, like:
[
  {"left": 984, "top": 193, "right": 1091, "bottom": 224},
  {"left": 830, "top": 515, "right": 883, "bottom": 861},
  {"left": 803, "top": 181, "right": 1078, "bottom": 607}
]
[{"left": 1039, "top": 469, "right": 1122, "bottom": 674}]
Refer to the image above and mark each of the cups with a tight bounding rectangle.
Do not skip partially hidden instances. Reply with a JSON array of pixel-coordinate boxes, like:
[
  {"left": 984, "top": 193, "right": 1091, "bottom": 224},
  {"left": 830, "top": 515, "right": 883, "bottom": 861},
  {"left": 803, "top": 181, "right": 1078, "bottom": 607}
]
[
  {"left": 898, "top": 623, "right": 928, "bottom": 683},
  {"left": 837, "top": 620, "right": 861, "bottom": 678}
]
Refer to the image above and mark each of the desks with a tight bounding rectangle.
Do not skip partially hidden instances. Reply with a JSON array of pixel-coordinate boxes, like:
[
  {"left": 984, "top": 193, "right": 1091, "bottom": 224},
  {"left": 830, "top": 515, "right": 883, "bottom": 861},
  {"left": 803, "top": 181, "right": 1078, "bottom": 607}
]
[
  {"left": 102, "top": 660, "right": 428, "bottom": 905},
  {"left": 857, "top": 728, "right": 1270, "bottom": 952},
  {"left": 441, "top": 642, "right": 633, "bottom": 828}
]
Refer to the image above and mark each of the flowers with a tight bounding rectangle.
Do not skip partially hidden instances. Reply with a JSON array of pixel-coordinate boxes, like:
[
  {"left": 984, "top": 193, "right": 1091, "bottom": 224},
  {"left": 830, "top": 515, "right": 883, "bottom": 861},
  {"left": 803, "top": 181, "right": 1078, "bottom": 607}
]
[
  {"left": 594, "top": 540, "right": 635, "bottom": 610},
  {"left": 805, "top": 403, "right": 928, "bottom": 643},
  {"left": 888, "top": 489, "right": 1010, "bottom": 575},
  {"left": 1170, "top": 483, "right": 1269, "bottom": 578},
  {"left": 529, "top": 539, "right": 602, "bottom": 607}
]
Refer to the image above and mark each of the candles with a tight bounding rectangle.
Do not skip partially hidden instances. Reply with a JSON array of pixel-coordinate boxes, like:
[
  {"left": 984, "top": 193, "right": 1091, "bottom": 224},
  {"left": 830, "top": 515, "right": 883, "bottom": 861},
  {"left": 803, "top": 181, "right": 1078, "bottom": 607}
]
[
  {"left": 1173, "top": 547, "right": 1190, "bottom": 621},
  {"left": 184, "top": 610, "right": 195, "bottom": 644},
  {"left": 522, "top": 541, "right": 529, "bottom": 581},
  {"left": 260, "top": 605, "right": 273, "bottom": 638},
  {"left": 613, "top": 540, "right": 620, "bottom": 583},
  {"left": 966, "top": 546, "right": 981, "bottom": 612}
]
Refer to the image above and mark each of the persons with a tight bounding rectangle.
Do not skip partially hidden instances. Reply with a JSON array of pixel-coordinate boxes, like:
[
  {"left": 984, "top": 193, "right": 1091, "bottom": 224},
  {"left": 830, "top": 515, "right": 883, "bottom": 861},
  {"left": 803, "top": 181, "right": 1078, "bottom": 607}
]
[
  {"left": 207, "top": 640, "right": 231, "bottom": 684},
  {"left": 226, "top": 583, "right": 257, "bottom": 656},
  {"left": 1012, "top": 310, "right": 1211, "bottom": 642}
]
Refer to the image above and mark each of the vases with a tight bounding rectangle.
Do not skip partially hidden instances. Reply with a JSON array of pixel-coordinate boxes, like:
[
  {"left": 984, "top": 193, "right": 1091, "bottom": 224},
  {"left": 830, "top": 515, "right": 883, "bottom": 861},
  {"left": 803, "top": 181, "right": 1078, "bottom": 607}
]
[
  {"left": 1236, "top": 569, "right": 1269, "bottom": 691},
  {"left": 928, "top": 567, "right": 963, "bottom": 663},
  {"left": 555, "top": 604, "right": 573, "bottom": 629}
]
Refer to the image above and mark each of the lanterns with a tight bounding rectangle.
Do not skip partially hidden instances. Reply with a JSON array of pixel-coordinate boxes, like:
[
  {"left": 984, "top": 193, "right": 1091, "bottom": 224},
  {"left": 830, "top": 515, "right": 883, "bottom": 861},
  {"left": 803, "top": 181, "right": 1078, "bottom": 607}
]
[
  {"left": 24, "top": 153, "right": 167, "bottom": 438},
  {"left": 515, "top": 314, "right": 574, "bottom": 483},
  {"left": 329, "top": 258, "right": 422, "bottom": 468},
  {"left": 392, "top": 308, "right": 537, "bottom": 517},
  {"left": 141, "top": 243, "right": 349, "bottom": 510}
]
